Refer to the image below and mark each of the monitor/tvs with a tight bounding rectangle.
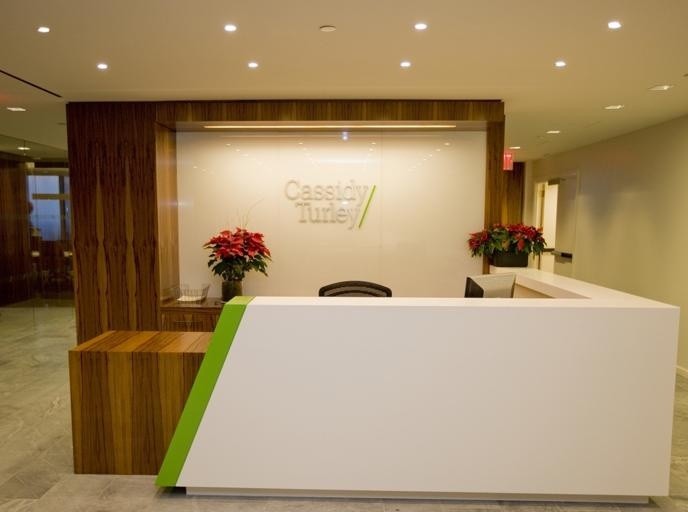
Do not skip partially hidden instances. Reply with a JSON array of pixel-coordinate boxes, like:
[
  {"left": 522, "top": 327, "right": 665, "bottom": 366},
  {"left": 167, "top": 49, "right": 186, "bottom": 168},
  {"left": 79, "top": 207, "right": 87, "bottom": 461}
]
[{"left": 464, "top": 271, "right": 515, "bottom": 298}]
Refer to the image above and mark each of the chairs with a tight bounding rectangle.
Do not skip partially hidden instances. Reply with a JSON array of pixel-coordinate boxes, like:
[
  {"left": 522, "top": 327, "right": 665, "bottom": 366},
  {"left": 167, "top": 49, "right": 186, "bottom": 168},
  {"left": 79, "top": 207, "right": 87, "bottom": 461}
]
[
  {"left": 30, "top": 236, "right": 73, "bottom": 297},
  {"left": 319, "top": 280, "right": 391, "bottom": 297}
]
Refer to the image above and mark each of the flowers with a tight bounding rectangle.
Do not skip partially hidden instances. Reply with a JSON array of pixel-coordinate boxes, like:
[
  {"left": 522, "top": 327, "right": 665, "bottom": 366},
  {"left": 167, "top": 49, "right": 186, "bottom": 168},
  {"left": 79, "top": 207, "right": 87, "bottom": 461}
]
[
  {"left": 465, "top": 222, "right": 547, "bottom": 260},
  {"left": 202, "top": 226, "right": 273, "bottom": 282}
]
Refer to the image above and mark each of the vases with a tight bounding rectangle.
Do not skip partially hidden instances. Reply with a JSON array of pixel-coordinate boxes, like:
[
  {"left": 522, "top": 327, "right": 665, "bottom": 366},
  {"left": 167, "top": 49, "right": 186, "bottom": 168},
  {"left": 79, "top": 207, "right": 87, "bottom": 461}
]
[
  {"left": 487, "top": 241, "right": 528, "bottom": 267},
  {"left": 220, "top": 278, "right": 244, "bottom": 304}
]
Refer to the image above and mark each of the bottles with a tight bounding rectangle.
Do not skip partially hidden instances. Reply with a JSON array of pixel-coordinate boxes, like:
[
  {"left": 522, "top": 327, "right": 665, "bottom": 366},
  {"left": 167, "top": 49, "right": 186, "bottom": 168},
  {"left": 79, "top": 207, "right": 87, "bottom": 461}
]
[{"left": 29, "top": 225, "right": 43, "bottom": 256}]
